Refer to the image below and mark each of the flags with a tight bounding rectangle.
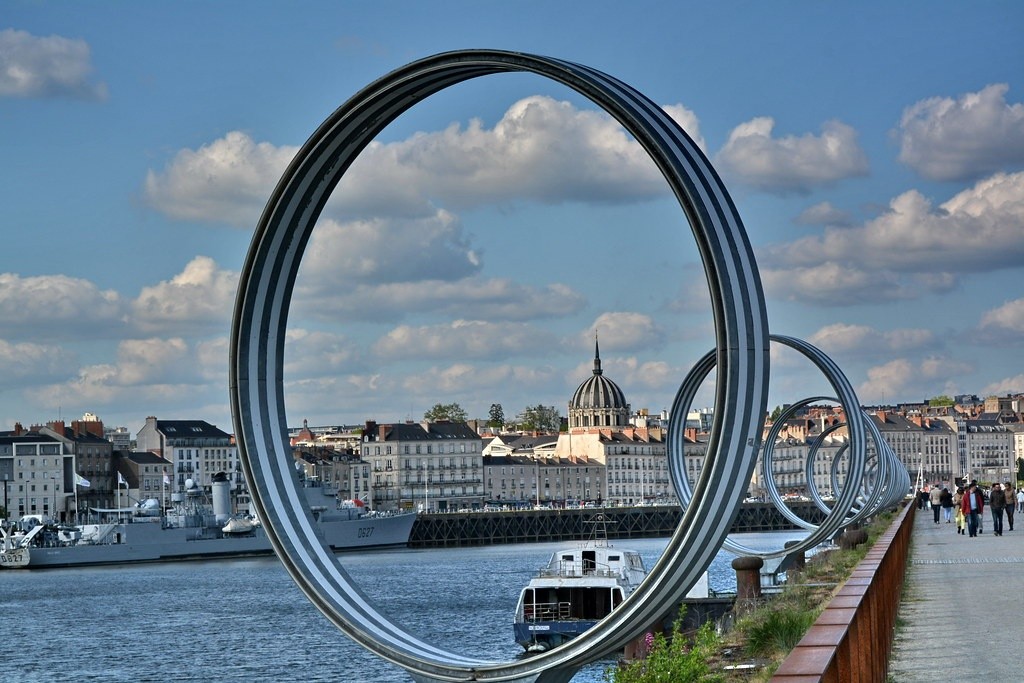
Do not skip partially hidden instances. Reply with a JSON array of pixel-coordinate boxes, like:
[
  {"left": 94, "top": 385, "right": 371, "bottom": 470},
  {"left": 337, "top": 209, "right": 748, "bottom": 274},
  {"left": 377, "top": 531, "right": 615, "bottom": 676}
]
[
  {"left": 118, "top": 473, "right": 129, "bottom": 489},
  {"left": 75, "top": 473, "right": 90, "bottom": 487},
  {"left": 163, "top": 470, "right": 170, "bottom": 484}
]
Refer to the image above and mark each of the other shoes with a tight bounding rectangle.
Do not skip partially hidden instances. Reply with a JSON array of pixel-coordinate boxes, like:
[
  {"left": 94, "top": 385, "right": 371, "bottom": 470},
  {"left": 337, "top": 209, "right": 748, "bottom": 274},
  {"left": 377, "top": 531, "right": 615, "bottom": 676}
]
[
  {"left": 1000, "top": 534, "right": 1002, "bottom": 536},
  {"left": 974, "top": 534, "right": 977, "bottom": 537},
  {"left": 1009, "top": 528, "right": 1014, "bottom": 530},
  {"left": 970, "top": 535, "right": 973, "bottom": 537},
  {"left": 948, "top": 519, "right": 950, "bottom": 523},
  {"left": 957, "top": 526, "right": 961, "bottom": 533},
  {"left": 945, "top": 520, "right": 948, "bottom": 523},
  {"left": 979, "top": 529, "right": 982, "bottom": 533},
  {"left": 994, "top": 532, "right": 998, "bottom": 536},
  {"left": 962, "top": 530, "right": 965, "bottom": 534}
]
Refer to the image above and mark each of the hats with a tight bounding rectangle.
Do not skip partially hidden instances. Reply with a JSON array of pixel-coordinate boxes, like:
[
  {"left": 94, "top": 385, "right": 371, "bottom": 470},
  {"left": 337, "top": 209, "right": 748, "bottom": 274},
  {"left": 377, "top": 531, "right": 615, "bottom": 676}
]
[{"left": 957, "top": 487, "right": 965, "bottom": 494}]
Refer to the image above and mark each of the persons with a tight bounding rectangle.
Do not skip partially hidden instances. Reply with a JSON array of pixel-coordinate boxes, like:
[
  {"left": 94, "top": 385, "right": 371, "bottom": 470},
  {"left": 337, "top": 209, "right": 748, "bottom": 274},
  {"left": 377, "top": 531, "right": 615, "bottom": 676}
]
[{"left": 914, "top": 479, "right": 1024, "bottom": 537}]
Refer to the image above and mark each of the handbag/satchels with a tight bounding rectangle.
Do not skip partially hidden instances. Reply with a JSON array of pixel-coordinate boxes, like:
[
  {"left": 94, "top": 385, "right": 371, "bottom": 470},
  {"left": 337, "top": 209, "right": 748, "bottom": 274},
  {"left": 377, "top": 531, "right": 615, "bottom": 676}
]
[{"left": 927, "top": 500, "right": 931, "bottom": 508}]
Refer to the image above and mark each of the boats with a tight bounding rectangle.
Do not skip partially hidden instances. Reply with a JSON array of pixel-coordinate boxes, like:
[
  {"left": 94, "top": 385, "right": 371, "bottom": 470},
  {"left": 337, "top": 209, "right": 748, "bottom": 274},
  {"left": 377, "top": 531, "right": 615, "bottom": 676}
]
[
  {"left": 0, "top": 468, "right": 421, "bottom": 570},
  {"left": 513, "top": 506, "right": 647, "bottom": 655}
]
